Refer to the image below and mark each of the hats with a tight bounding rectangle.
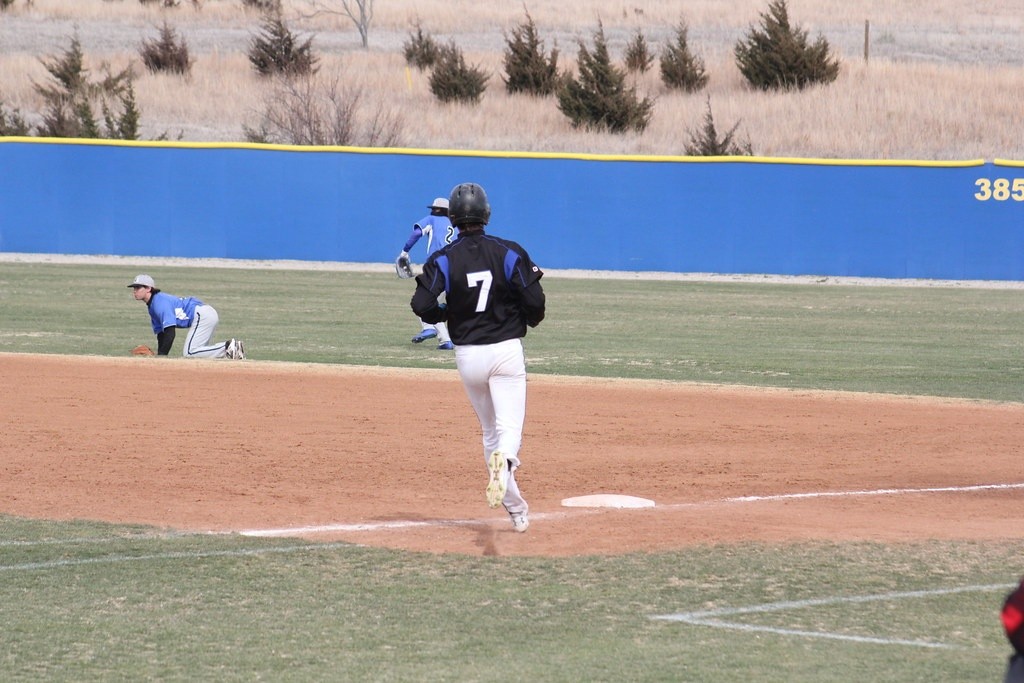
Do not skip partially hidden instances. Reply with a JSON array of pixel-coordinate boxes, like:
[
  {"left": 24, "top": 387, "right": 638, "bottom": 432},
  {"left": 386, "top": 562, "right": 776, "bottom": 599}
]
[
  {"left": 127, "top": 275, "right": 154, "bottom": 288},
  {"left": 427, "top": 198, "right": 449, "bottom": 209}
]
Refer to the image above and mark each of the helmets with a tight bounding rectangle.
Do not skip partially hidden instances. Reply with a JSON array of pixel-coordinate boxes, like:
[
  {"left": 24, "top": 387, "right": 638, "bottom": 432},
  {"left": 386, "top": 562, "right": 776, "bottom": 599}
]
[{"left": 448, "top": 182, "right": 488, "bottom": 227}]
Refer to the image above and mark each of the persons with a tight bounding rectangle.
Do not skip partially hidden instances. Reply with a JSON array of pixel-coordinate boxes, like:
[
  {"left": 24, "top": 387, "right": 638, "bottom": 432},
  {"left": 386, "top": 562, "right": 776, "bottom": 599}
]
[
  {"left": 395, "top": 197, "right": 459, "bottom": 349},
  {"left": 128, "top": 274, "right": 246, "bottom": 359},
  {"left": 409, "top": 182, "right": 546, "bottom": 531}
]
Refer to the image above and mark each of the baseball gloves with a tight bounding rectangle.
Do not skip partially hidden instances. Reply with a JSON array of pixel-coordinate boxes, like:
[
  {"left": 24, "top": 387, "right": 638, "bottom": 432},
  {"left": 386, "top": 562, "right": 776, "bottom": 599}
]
[
  {"left": 395, "top": 251, "right": 416, "bottom": 280},
  {"left": 132, "top": 344, "right": 154, "bottom": 356}
]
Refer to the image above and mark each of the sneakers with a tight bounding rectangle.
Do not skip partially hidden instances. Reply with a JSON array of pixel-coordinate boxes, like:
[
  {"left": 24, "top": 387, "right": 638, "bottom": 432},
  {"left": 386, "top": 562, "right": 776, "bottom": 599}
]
[
  {"left": 236, "top": 341, "right": 244, "bottom": 359},
  {"left": 412, "top": 329, "right": 438, "bottom": 343},
  {"left": 437, "top": 342, "right": 453, "bottom": 349},
  {"left": 485, "top": 452, "right": 508, "bottom": 509},
  {"left": 226, "top": 338, "right": 236, "bottom": 359},
  {"left": 513, "top": 515, "right": 529, "bottom": 532}
]
